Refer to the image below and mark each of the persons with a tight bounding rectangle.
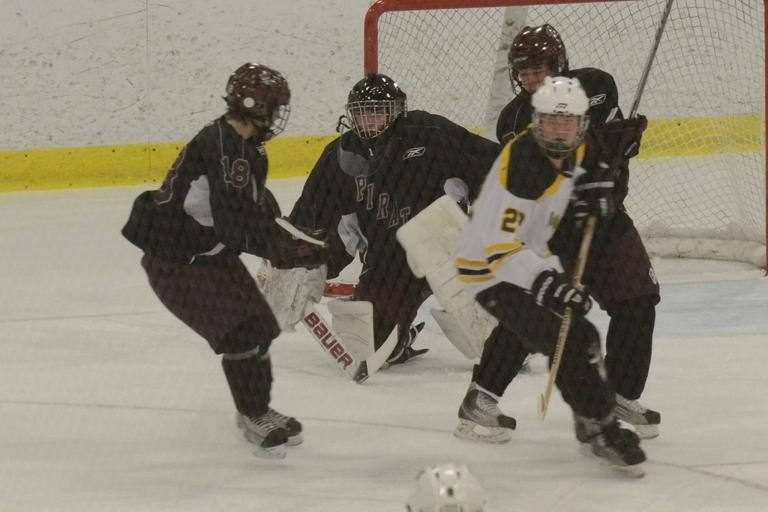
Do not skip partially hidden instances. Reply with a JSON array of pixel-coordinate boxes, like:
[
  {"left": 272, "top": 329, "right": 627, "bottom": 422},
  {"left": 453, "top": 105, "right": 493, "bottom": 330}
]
[
  {"left": 260, "top": 74, "right": 533, "bottom": 386},
  {"left": 446, "top": 75, "right": 650, "bottom": 469},
  {"left": 495, "top": 24, "right": 660, "bottom": 426},
  {"left": 120, "top": 58, "right": 340, "bottom": 449}
]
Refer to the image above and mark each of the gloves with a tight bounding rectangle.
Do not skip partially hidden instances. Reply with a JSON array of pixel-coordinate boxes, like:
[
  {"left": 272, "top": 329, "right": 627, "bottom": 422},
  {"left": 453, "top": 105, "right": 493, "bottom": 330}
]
[
  {"left": 528, "top": 270, "right": 596, "bottom": 325},
  {"left": 267, "top": 215, "right": 335, "bottom": 270},
  {"left": 598, "top": 115, "right": 647, "bottom": 162},
  {"left": 577, "top": 165, "right": 629, "bottom": 220}
]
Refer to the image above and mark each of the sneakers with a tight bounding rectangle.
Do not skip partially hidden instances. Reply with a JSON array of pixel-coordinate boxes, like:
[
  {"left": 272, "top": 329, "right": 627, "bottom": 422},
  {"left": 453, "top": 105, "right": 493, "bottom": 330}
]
[
  {"left": 457, "top": 386, "right": 516, "bottom": 429},
  {"left": 236, "top": 405, "right": 303, "bottom": 451},
  {"left": 573, "top": 412, "right": 646, "bottom": 469},
  {"left": 611, "top": 390, "right": 661, "bottom": 426}
]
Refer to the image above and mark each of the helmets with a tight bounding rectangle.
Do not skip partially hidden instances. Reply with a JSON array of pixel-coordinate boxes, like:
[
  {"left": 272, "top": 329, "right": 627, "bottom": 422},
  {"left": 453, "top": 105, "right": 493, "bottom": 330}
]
[
  {"left": 346, "top": 74, "right": 408, "bottom": 152},
  {"left": 226, "top": 62, "right": 291, "bottom": 143},
  {"left": 506, "top": 24, "right": 570, "bottom": 95},
  {"left": 403, "top": 466, "right": 484, "bottom": 511},
  {"left": 530, "top": 76, "right": 590, "bottom": 161}
]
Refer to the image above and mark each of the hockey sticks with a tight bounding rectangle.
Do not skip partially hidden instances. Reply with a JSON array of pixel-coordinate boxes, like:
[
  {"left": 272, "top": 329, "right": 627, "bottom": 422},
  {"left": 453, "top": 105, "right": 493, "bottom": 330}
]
[
  {"left": 300, "top": 304, "right": 403, "bottom": 384},
  {"left": 541, "top": 0, "right": 674, "bottom": 426}
]
